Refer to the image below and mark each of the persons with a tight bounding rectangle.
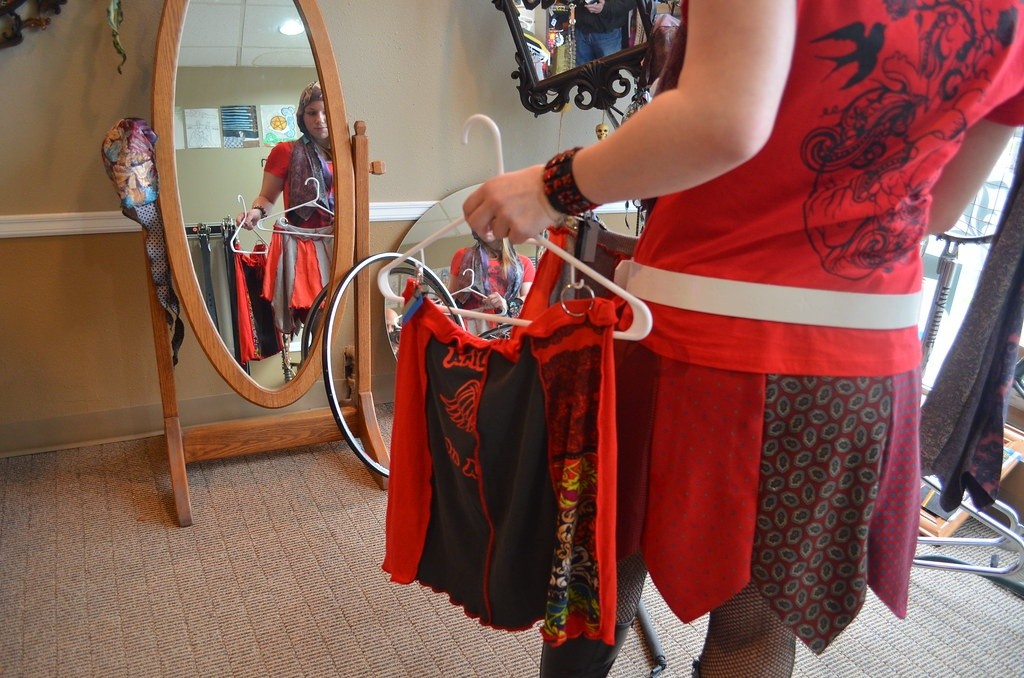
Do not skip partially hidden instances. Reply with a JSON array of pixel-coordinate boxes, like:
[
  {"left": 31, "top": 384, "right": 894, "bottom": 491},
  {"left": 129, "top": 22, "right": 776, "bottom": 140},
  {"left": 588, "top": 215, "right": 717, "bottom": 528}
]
[
  {"left": 441, "top": 230, "right": 536, "bottom": 344},
  {"left": 561, "top": 0, "right": 640, "bottom": 68},
  {"left": 237, "top": 80, "right": 339, "bottom": 352},
  {"left": 463, "top": 0, "right": 1024, "bottom": 678}
]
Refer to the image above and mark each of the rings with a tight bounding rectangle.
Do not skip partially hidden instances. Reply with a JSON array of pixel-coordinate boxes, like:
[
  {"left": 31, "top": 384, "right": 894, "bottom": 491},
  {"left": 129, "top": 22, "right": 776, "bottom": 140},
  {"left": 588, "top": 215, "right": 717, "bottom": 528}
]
[{"left": 482, "top": 225, "right": 499, "bottom": 243}]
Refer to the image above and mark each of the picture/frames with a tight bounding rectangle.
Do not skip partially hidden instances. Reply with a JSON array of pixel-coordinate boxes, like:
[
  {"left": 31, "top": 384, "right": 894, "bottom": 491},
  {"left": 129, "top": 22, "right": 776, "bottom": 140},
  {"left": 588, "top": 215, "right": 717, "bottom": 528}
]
[{"left": 919, "top": 448, "right": 1021, "bottom": 539}]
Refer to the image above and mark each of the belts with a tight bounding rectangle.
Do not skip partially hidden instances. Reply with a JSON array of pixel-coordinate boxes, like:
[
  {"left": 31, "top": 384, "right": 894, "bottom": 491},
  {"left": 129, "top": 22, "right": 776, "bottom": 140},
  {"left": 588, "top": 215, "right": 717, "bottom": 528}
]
[{"left": 614, "top": 259, "right": 922, "bottom": 329}]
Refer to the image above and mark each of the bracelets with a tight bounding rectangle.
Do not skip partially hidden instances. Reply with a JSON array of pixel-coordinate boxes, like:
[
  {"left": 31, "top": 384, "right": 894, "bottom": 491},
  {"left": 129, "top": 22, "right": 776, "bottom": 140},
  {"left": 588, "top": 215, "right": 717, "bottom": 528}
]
[
  {"left": 252, "top": 205, "right": 268, "bottom": 221},
  {"left": 543, "top": 145, "right": 602, "bottom": 217}
]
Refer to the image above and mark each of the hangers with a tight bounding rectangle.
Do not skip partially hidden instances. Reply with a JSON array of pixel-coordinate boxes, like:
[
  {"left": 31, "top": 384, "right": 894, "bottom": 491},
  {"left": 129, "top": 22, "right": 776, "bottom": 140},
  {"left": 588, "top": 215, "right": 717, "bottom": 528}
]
[
  {"left": 256, "top": 176, "right": 335, "bottom": 238},
  {"left": 394, "top": 267, "right": 506, "bottom": 330},
  {"left": 377, "top": 113, "right": 652, "bottom": 342},
  {"left": 230, "top": 195, "right": 269, "bottom": 254}
]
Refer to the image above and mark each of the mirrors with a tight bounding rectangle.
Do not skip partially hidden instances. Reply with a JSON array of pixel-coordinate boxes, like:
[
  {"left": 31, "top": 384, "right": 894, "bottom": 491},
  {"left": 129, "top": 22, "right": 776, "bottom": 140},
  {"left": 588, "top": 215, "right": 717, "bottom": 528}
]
[
  {"left": 384, "top": 184, "right": 549, "bottom": 364},
  {"left": 100, "top": 0, "right": 387, "bottom": 527},
  {"left": 489, "top": 0, "right": 654, "bottom": 118}
]
